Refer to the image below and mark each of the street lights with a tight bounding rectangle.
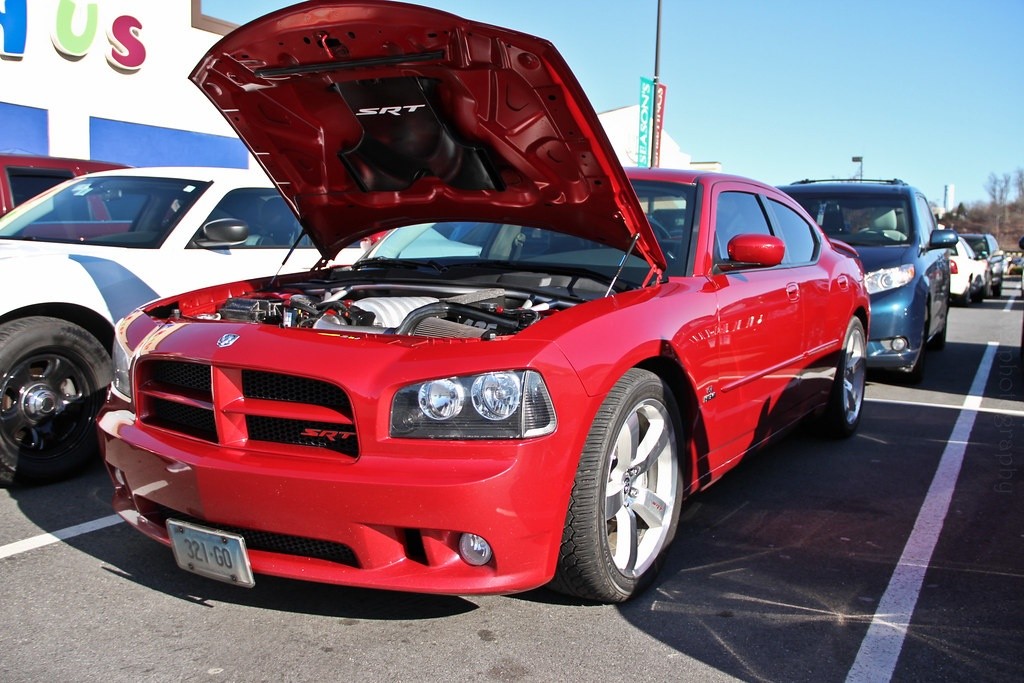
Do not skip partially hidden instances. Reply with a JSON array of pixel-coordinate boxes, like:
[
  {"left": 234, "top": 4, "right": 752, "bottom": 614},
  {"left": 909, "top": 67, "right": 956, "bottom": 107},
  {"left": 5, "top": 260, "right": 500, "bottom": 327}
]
[{"left": 852, "top": 157, "right": 862, "bottom": 181}]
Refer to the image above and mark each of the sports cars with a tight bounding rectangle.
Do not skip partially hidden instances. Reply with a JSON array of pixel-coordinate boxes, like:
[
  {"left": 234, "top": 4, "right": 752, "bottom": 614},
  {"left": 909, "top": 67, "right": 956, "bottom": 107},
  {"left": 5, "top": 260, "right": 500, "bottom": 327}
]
[{"left": 94, "top": 0, "right": 870, "bottom": 607}]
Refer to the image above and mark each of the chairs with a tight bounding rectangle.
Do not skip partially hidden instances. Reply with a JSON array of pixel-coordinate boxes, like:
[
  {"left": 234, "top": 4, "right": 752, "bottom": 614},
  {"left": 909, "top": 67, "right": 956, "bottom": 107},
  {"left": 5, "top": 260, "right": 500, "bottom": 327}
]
[
  {"left": 256, "top": 197, "right": 306, "bottom": 247},
  {"left": 652, "top": 209, "right": 686, "bottom": 269},
  {"left": 856, "top": 205, "right": 908, "bottom": 241}
]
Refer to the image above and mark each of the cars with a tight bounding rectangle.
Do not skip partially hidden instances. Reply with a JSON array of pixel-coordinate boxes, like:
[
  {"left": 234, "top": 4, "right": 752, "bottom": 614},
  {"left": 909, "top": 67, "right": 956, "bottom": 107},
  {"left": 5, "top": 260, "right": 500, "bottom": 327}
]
[
  {"left": 0, "top": 166, "right": 483, "bottom": 491},
  {"left": 770, "top": 179, "right": 960, "bottom": 377},
  {"left": 950, "top": 236, "right": 989, "bottom": 305},
  {"left": 962, "top": 233, "right": 1004, "bottom": 298},
  {"left": 0, "top": 154, "right": 179, "bottom": 240}
]
